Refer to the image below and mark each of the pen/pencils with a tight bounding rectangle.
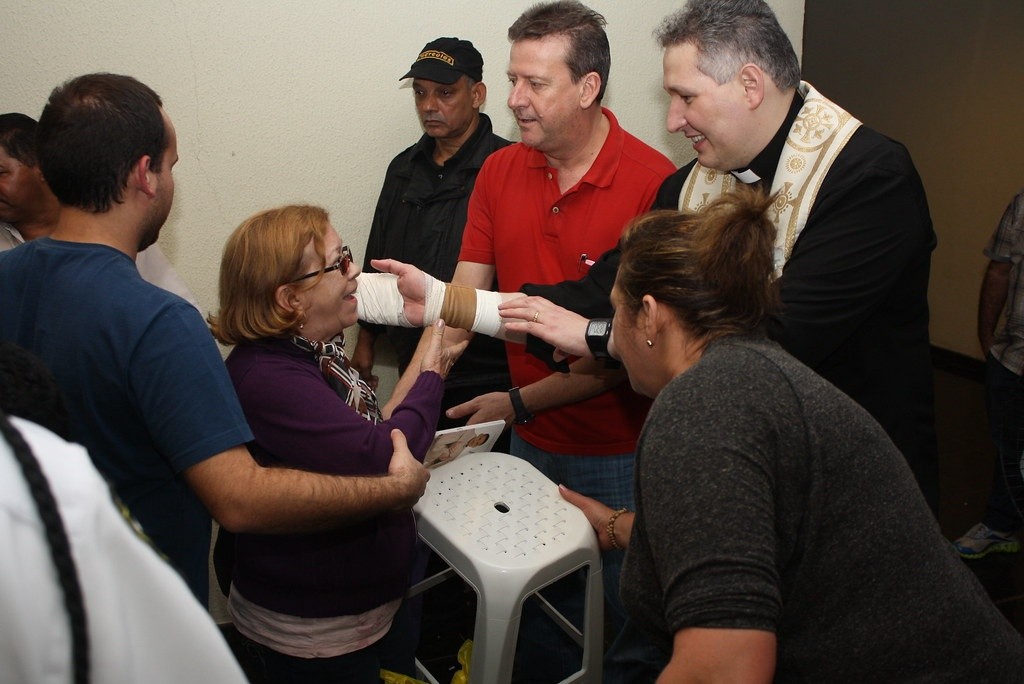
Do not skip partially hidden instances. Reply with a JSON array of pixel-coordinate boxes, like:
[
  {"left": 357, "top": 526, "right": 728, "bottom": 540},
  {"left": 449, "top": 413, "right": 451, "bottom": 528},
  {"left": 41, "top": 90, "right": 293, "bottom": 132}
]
[{"left": 577, "top": 253, "right": 588, "bottom": 272}]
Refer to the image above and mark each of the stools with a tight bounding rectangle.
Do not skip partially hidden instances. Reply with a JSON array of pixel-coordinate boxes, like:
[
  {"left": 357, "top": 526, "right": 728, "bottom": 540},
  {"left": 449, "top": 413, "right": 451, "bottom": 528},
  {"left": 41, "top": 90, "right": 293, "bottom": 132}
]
[{"left": 400, "top": 451, "right": 604, "bottom": 684}]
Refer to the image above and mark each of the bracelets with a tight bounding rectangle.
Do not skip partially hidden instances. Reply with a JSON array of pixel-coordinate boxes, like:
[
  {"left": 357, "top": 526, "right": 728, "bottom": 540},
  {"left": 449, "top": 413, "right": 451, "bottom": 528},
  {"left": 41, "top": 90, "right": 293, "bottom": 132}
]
[{"left": 606, "top": 507, "right": 627, "bottom": 550}]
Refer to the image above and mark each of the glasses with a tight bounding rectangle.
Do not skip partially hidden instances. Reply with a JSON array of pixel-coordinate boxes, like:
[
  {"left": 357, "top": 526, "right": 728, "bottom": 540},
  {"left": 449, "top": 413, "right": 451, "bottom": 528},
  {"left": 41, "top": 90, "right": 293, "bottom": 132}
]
[{"left": 285, "top": 246, "right": 353, "bottom": 285}]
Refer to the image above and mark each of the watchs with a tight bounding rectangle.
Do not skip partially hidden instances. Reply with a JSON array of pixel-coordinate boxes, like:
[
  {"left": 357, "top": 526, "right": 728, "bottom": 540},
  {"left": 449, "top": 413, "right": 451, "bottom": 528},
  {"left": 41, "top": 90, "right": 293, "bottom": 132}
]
[
  {"left": 585, "top": 318, "right": 612, "bottom": 360},
  {"left": 508, "top": 386, "right": 535, "bottom": 426}
]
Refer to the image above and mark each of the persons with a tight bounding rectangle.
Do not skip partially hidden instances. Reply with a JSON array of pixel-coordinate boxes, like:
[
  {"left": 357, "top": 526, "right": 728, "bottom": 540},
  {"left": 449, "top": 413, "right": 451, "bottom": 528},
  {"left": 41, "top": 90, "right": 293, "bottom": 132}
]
[
  {"left": 0, "top": 71, "right": 430, "bottom": 605},
  {"left": 950, "top": 189, "right": 1024, "bottom": 558},
  {"left": 558, "top": 187, "right": 1024, "bottom": 684},
  {"left": 378, "top": 0, "right": 678, "bottom": 684},
  {"left": 204, "top": 204, "right": 469, "bottom": 684},
  {"left": 0, "top": 113, "right": 202, "bottom": 311},
  {"left": 422, "top": 428, "right": 490, "bottom": 469},
  {"left": 351, "top": 38, "right": 517, "bottom": 454},
  {"left": 352, "top": 0, "right": 940, "bottom": 521},
  {"left": 0, "top": 413, "right": 250, "bottom": 683}
]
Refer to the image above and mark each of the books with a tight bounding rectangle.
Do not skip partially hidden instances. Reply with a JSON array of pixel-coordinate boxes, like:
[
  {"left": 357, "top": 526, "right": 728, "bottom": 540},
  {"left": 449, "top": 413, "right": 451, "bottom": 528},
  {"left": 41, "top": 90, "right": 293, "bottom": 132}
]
[{"left": 422, "top": 420, "right": 506, "bottom": 472}]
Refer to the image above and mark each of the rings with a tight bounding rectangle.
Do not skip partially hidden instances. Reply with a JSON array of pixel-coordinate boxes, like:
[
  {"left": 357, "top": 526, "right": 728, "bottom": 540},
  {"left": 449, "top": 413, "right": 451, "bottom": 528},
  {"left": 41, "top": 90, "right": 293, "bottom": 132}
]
[{"left": 534, "top": 312, "right": 539, "bottom": 323}]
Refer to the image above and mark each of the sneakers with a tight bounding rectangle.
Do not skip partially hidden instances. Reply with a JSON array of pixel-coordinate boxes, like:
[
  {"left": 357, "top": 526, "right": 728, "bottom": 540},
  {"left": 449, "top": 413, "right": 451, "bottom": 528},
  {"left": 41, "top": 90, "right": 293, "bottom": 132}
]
[{"left": 954, "top": 520, "right": 1021, "bottom": 558}]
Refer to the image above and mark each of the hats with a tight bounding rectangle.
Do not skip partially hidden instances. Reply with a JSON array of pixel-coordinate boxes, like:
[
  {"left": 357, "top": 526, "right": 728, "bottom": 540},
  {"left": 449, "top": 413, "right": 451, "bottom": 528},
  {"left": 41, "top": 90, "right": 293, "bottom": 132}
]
[{"left": 399, "top": 37, "right": 484, "bottom": 83}]
